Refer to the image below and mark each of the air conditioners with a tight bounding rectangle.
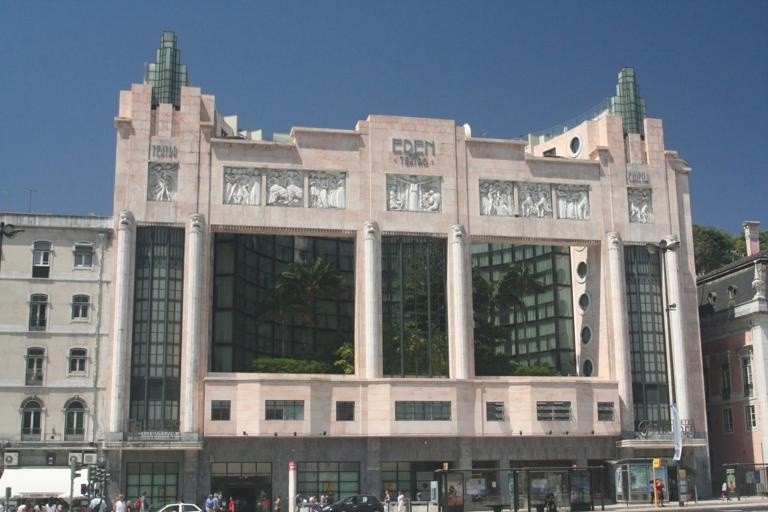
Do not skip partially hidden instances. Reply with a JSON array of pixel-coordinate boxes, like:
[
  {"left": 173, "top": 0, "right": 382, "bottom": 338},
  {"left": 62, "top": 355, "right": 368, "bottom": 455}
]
[
  {"left": 4, "top": 452, "right": 19, "bottom": 465},
  {"left": 68, "top": 452, "right": 83, "bottom": 467},
  {"left": 83, "top": 453, "right": 98, "bottom": 465}
]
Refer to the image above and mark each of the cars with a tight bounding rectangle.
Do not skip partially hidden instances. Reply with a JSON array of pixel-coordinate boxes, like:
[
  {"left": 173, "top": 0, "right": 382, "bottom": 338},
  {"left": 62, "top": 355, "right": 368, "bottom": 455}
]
[
  {"left": 158, "top": 503, "right": 202, "bottom": 511},
  {"left": 321, "top": 495, "right": 383, "bottom": 511}
]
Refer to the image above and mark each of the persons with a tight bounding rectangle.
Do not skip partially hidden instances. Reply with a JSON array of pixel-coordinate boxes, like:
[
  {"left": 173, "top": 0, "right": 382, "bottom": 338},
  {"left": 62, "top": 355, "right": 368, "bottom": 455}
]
[
  {"left": 266, "top": 171, "right": 287, "bottom": 206},
  {"left": 648, "top": 480, "right": 653, "bottom": 505},
  {"left": 383, "top": 490, "right": 393, "bottom": 512},
  {"left": 655, "top": 478, "right": 665, "bottom": 507},
  {"left": 479, "top": 179, "right": 589, "bottom": 220},
  {"left": 391, "top": 174, "right": 436, "bottom": 212},
  {"left": 161, "top": 163, "right": 177, "bottom": 201},
  {"left": 150, "top": 165, "right": 171, "bottom": 201},
  {"left": 223, "top": 167, "right": 261, "bottom": 206},
  {"left": 628, "top": 188, "right": 641, "bottom": 222},
  {"left": 423, "top": 189, "right": 440, "bottom": 212},
  {"left": 637, "top": 190, "right": 650, "bottom": 224},
  {"left": 308, "top": 171, "right": 345, "bottom": 209},
  {"left": 281, "top": 171, "right": 303, "bottom": 206},
  {"left": 204, "top": 491, "right": 329, "bottom": 512},
  {"left": 544, "top": 493, "right": 556, "bottom": 512},
  {"left": 387, "top": 185, "right": 403, "bottom": 210},
  {"left": 414, "top": 488, "right": 422, "bottom": 501},
  {"left": 396, "top": 490, "right": 406, "bottom": 512},
  {"left": 720, "top": 480, "right": 727, "bottom": 501},
  {"left": 653, "top": 479, "right": 660, "bottom": 505},
  {"left": 14, "top": 491, "right": 151, "bottom": 512}
]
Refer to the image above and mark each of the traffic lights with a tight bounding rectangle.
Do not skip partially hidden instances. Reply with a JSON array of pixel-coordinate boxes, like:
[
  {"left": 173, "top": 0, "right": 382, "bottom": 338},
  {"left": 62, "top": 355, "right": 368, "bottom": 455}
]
[
  {"left": 70, "top": 461, "right": 81, "bottom": 479},
  {"left": 87, "top": 464, "right": 111, "bottom": 483}
]
